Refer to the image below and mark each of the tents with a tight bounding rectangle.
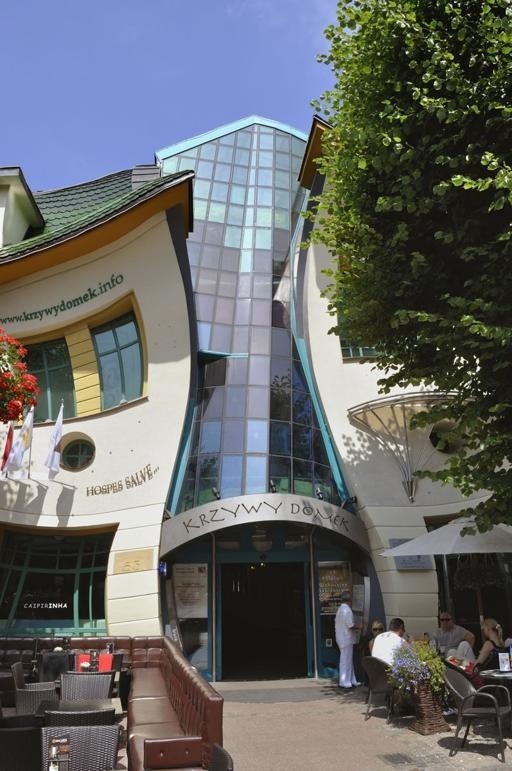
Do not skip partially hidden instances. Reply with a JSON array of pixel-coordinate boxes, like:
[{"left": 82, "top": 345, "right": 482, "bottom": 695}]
[{"left": 1, "top": 419, "right": 15, "bottom": 474}]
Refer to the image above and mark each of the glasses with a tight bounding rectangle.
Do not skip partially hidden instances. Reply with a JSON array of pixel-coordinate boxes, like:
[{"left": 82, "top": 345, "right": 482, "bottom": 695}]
[
  {"left": 439, "top": 619, "right": 451, "bottom": 622},
  {"left": 372, "top": 628, "right": 384, "bottom": 631}
]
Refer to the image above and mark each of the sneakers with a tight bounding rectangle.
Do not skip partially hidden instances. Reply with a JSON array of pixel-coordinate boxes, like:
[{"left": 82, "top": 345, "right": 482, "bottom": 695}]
[{"left": 338, "top": 681, "right": 362, "bottom": 689}]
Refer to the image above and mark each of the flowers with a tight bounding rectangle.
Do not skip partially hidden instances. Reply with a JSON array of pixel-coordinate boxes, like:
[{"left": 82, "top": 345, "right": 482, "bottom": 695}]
[{"left": 0, "top": 328, "right": 40, "bottom": 424}]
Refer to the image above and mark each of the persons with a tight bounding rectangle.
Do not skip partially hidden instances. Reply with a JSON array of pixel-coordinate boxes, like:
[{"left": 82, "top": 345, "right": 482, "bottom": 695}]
[
  {"left": 333, "top": 591, "right": 363, "bottom": 690},
  {"left": 470, "top": 618, "right": 508, "bottom": 672},
  {"left": 369, "top": 619, "right": 383, "bottom": 653},
  {"left": 432, "top": 610, "right": 475, "bottom": 656},
  {"left": 371, "top": 618, "right": 457, "bottom": 715}
]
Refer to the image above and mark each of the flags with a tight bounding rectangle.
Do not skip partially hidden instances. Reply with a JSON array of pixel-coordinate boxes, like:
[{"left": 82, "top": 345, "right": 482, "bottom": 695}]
[
  {"left": 6, "top": 406, "right": 33, "bottom": 478},
  {"left": 44, "top": 404, "right": 62, "bottom": 480}
]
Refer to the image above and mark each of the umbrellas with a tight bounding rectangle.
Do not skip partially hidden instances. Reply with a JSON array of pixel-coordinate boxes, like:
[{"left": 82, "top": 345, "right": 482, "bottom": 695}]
[{"left": 377, "top": 511, "right": 511, "bottom": 644}]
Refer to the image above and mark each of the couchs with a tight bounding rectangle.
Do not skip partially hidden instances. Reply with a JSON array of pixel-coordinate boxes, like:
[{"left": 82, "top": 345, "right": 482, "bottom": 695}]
[
  {"left": 2, "top": 636, "right": 134, "bottom": 691},
  {"left": 127, "top": 633, "right": 224, "bottom": 770}
]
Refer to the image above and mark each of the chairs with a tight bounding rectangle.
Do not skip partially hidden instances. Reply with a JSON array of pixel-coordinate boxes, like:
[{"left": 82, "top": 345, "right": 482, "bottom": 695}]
[
  {"left": 0, "top": 650, "right": 132, "bottom": 771},
  {"left": 359, "top": 633, "right": 511, "bottom": 763},
  {"left": 207, "top": 743, "right": 234, "bottom": 771}
]
